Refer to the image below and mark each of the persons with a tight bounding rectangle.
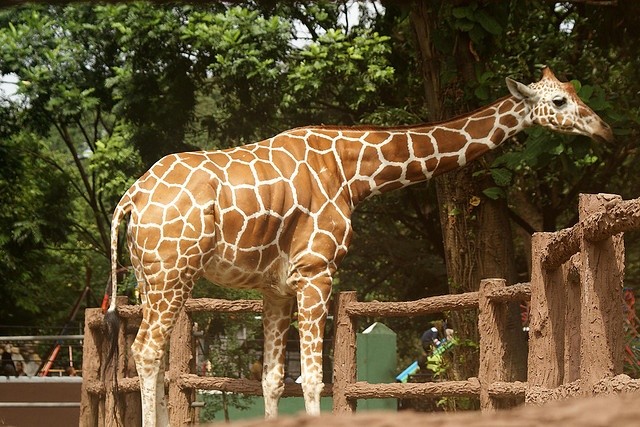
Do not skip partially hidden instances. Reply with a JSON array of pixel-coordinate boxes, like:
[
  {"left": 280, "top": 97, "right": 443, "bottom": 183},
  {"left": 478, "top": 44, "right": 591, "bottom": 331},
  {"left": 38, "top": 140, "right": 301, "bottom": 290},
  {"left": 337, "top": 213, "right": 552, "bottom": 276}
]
[
  {"left": 0, "top": 335, "right": 43, "bottom": 377},
  {"left": 66, "top": 366, "right": 76, "bottom": 376},
  {"left": 253, "top": 354, "right": 302, "bottom": 384},
  {"left": 416, "top": 318, "right": 455, "bottom": 373}
]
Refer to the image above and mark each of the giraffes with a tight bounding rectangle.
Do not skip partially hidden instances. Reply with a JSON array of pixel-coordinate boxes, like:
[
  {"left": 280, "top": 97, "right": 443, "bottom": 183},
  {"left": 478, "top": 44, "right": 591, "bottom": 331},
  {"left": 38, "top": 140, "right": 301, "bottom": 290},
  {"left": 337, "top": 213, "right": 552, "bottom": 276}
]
[{"left": 102, "top": 65, "right": 614, "bottom": 427}]
